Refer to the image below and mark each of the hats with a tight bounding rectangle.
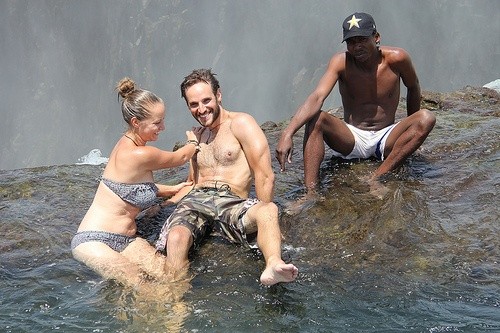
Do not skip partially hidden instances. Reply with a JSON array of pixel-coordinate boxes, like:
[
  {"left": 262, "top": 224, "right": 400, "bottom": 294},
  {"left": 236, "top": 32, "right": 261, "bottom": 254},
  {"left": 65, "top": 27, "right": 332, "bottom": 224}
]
[{"left": 341, "top": 12, "right": 375, "bottom": 43}]
